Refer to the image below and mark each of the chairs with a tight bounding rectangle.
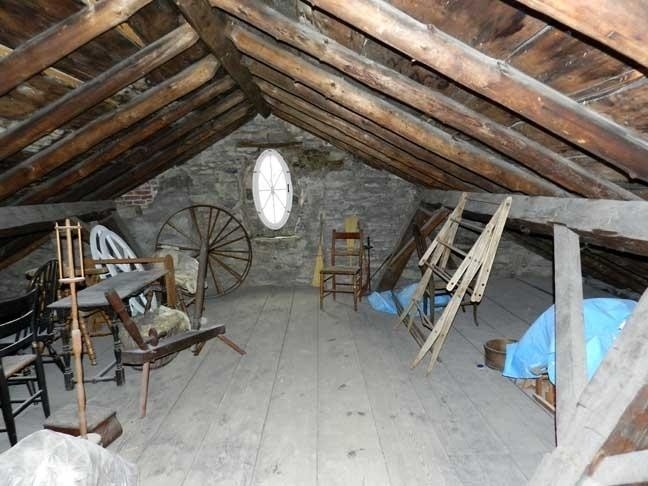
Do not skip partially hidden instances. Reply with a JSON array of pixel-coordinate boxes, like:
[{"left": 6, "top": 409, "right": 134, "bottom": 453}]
[
  {"left": 413, "top": 224, "right": 483, "bottom": 326},
  {"left": 320, "top": 229, "right": 364, "bottom": 312},
  {"left": 0, "top": 258, "right": 66, "bottom": 447}
]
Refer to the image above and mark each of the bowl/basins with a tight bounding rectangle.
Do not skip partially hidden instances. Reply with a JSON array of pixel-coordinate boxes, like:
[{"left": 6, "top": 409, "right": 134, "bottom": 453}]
[{"left": 483, "top": 337, "right": 521, "bottom": 373}]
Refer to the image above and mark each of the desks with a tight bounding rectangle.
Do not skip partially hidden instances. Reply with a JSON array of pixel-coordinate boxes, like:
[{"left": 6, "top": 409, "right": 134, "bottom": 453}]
[{"left": 45, "top": 268, "right": 170, "bottom": 391}]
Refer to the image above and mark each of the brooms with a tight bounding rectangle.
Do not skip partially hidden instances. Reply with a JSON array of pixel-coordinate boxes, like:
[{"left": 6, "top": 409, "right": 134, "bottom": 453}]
[
  {"left": 309, "top": 213, "right": 327, "bottom": 288},
  {"left": 343, "top": 215, "right": 360, "bottom": 281}
]
